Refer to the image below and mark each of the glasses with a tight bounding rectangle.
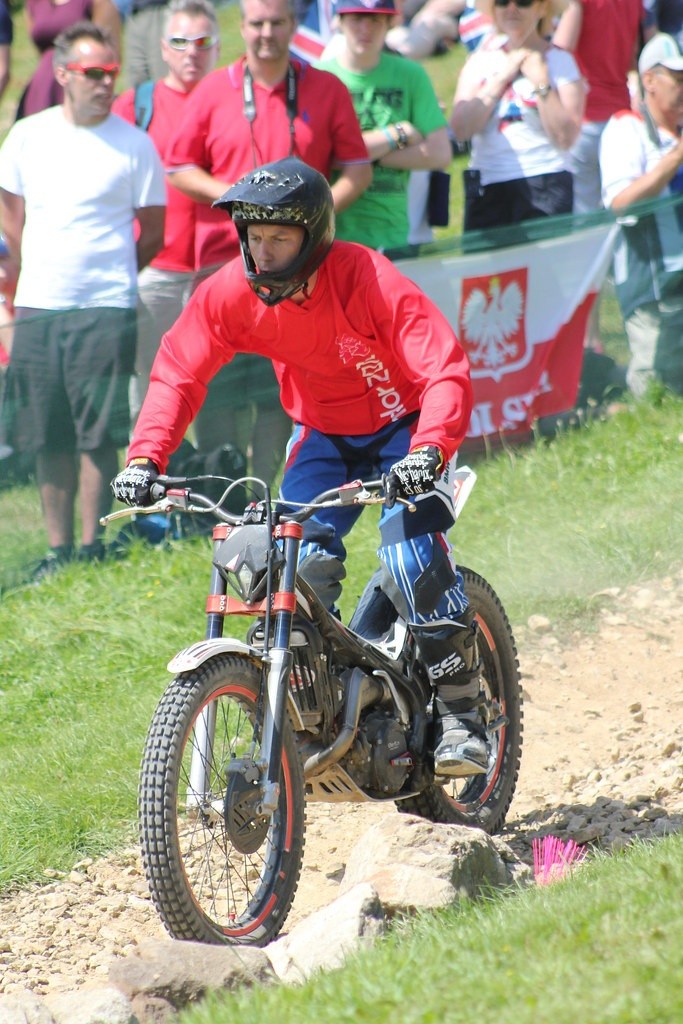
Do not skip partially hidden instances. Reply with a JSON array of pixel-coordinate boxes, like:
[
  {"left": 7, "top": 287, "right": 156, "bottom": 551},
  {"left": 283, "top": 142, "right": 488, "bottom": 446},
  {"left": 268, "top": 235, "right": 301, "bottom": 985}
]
[
  {"left": 494, "top": 0, "right": 531, "bottom": 8},
  {"left": 166, "top": 33, "right": 218, "bottom": 51},
  {"left": 66, "top": 63, "right": 121, "bottom": 81}
]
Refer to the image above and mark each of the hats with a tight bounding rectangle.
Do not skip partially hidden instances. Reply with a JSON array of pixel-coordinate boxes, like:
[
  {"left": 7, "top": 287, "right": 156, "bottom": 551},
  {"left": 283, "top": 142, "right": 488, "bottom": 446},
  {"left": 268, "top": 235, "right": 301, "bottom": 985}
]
[
  {"left": 638, "top": 32, "right": 683, "bottom": 76},
  {"left": 336, "top": 0, "right": 398, "bottom": 16}
]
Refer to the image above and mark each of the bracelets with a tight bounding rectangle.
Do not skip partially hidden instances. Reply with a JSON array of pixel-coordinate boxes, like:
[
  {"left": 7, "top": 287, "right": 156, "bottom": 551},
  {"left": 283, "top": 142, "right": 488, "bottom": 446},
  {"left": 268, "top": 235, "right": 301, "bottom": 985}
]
[{"left": 380, "top": 122, "right": 408, "bottom": 152}]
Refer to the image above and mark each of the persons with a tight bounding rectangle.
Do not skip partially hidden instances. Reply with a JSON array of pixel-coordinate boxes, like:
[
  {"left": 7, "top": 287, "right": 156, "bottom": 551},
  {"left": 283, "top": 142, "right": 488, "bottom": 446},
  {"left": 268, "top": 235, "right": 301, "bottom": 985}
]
[
  {"left": 0, "top": 21, "right": 168, "bottom": 579},
  {"left": 111, "top": 0, "right": 243, "bottom": 542},
  {"left": 0, "top": 0, "right": 178, "bottom": 368},
  {"left": 114, "top": 156, "right": 491, "bottom": 775},
  {"left": 597, "top": 33, "right": 683, "bottom": 414},
  {"left": 384, "top": 0, "right": 683, "bottom": 347},
  {"left": 310, "top": 0, "right": 452, "bottom": 261},
  {"left": 167, "top": 0, "right": 372, "bottom": 504},
  {"left": 448, "top": 0, "right": 587, "bottom": 256}
]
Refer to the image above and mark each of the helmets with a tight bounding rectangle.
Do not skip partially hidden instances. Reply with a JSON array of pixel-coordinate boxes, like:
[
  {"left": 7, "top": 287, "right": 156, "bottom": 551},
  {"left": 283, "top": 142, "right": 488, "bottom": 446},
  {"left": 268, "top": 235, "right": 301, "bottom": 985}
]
[{"left": 210, "top": 157, "right": 335, "bottom": 306}]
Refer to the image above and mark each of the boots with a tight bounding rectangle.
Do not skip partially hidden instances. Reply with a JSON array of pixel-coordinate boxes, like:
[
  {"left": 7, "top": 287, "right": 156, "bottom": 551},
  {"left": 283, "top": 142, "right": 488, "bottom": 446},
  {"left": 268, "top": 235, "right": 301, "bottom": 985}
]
[{"left": 410, "top": 605, "right": 493, "bottom": 778}]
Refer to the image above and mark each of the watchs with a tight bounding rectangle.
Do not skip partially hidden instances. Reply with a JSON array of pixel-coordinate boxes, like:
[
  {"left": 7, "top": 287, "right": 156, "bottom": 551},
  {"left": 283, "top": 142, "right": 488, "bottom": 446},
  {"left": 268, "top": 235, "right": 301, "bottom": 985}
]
[{"left": 533, "top": 83, "right": 551, "bottom": 98}]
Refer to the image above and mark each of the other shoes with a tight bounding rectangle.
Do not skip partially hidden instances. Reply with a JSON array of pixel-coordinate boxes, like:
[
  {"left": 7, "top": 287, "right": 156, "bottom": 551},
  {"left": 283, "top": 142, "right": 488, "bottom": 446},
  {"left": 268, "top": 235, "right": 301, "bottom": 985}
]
[
  {"left": 78, "top": 541, "right": 104, "bottom": 566},
  {"left": 29, "top": 547, "right": 73, "bottom": 582}
]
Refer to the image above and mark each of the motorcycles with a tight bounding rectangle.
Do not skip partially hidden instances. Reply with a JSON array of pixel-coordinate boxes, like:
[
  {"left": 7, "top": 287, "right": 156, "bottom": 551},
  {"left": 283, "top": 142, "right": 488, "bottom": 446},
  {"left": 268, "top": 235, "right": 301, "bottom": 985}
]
[{"left": 101, "top": 470, "right": 524, "bottom": 947}]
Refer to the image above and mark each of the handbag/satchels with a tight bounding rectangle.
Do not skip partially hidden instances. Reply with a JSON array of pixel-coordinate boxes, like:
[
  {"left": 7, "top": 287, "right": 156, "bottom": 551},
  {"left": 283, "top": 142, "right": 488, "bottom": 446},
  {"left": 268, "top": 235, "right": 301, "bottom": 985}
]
[{"left": 164, "top": 439, "right": 249, "bottom": 537}]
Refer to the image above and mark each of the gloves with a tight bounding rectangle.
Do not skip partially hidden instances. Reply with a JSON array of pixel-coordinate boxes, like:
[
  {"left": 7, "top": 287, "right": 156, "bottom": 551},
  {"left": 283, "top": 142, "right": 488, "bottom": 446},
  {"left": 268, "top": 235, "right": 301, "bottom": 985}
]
[
  {"left": 384, "top": 445, "right": 442, "bottom": 509},
  {"left": 109, "top": 459, "right": 160, "bottom": 515}
]
